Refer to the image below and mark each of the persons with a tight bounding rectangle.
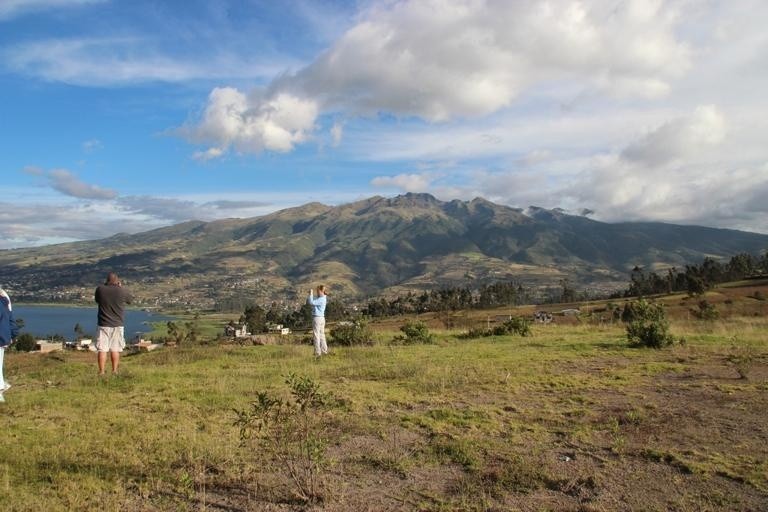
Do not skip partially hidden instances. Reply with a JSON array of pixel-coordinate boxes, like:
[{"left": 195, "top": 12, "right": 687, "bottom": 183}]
[
  {"left": 0, "top": 287, "right": 20, "bottom": 405},
  {"left": 306, "top": 285, "right": 329, "bottom": 356},
  {"left": 95, "top": 272, "right": 135, "bottom": 375}
]
